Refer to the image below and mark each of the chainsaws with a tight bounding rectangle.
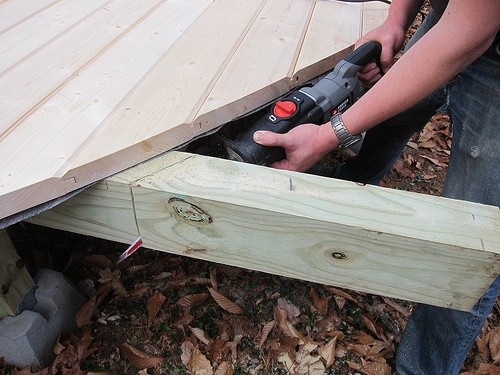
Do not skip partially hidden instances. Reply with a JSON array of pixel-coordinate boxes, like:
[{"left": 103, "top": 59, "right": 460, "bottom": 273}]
[{"left": 113, "top": 40, "right": 386, "bottom": 266}]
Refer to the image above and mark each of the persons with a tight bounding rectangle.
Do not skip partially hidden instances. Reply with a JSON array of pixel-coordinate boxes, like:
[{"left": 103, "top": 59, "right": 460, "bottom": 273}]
[{"left": 253, "top": 0, "right": 500, "bottom": 375}]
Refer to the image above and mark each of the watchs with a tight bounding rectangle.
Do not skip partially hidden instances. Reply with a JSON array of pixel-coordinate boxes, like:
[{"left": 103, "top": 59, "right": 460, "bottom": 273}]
[{"left": 330, "top": 112, "right": 362, "bottom": 151}]
